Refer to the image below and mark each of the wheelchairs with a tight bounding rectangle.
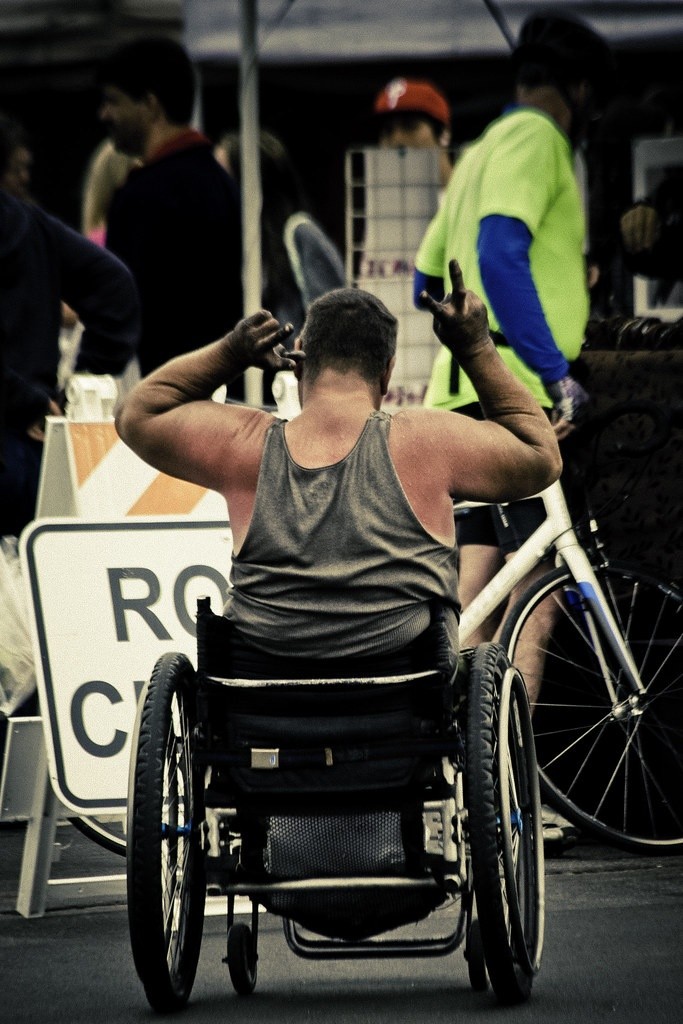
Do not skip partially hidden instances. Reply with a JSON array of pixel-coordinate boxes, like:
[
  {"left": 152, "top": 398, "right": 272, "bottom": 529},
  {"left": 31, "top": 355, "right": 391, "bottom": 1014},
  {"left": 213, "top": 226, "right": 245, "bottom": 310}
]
[{"left": 123, "top": 589, "right": 546, "bottom": 1016}]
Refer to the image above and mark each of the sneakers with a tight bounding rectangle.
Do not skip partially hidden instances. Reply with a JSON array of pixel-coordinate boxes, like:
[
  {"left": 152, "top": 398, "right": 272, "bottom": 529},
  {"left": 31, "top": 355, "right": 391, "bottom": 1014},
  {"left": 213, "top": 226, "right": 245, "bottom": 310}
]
[{"left": 512, "top": 802, "right": 582, "bottom": 852}]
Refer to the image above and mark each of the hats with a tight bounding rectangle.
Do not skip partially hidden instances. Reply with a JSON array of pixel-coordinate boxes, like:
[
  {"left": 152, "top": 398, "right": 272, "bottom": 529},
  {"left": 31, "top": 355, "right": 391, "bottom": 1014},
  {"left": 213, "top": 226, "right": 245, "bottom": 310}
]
[{"left": 375, "top": 79, "right": 449, "bottom": 128}]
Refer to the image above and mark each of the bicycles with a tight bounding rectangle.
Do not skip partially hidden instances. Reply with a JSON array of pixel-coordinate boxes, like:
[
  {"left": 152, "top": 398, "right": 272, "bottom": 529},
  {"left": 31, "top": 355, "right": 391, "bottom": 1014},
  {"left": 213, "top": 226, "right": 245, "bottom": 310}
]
[{"left": 61, "top": 383, "right": 683, "bottom": 864}]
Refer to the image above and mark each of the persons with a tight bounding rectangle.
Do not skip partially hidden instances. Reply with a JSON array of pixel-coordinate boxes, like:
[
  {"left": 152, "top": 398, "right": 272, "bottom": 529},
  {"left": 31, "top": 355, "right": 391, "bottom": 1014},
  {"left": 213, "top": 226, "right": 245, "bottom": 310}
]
[
  {"left": 1, "top": 12, "right": 683, "bottom": 858},
  {"left": 117, "top": 259, "right": 564, "bottom": 891}
]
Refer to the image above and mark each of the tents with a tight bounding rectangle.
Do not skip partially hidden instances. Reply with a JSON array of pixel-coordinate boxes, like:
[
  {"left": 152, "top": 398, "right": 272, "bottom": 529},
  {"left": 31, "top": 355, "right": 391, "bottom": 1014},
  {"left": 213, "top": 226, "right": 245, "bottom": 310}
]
[{"left": 181, "top": 0, "right": 683, "bottom": 139}]
